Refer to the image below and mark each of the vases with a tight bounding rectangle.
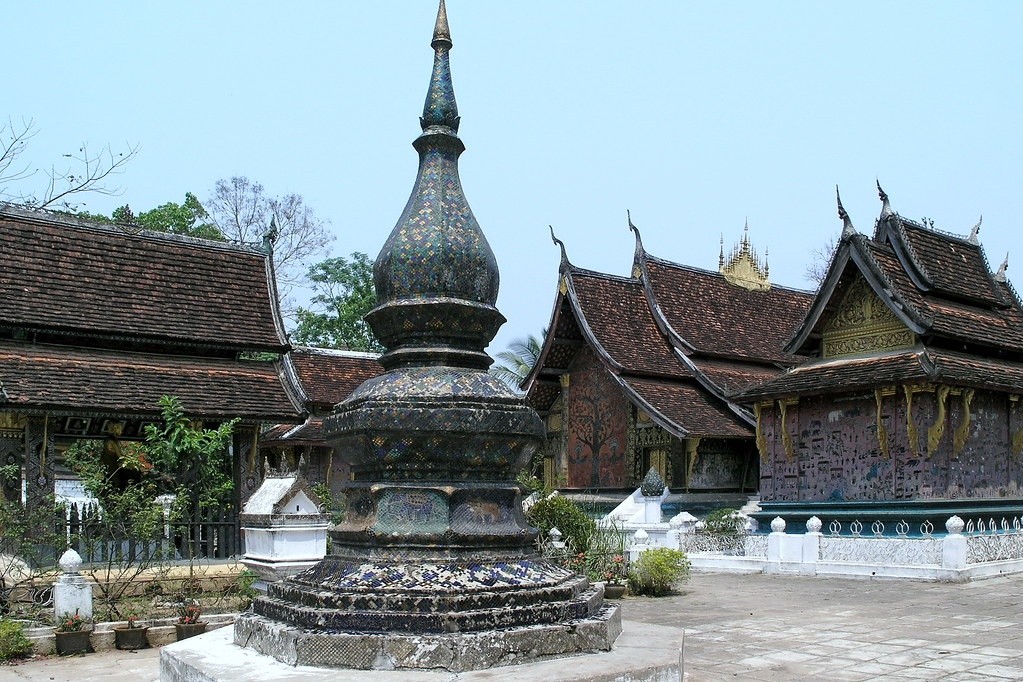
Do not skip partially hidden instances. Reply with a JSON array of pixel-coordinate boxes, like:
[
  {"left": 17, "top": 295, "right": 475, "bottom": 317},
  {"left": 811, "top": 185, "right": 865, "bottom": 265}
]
[
  {"left": 173, "top": 621, "right": 208, "bottom": 641},
  {"left": 112, "top": 625, "right": 149, "bottom": 649},
  {"left": 53, "top": 630, "right": 92, "bottom": 655},
  {"left": 603, "top": 584, "right": 626, "bottom": 599}
]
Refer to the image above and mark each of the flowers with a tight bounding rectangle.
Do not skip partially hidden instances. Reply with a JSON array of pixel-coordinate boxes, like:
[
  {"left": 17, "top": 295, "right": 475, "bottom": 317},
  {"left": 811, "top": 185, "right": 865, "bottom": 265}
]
[
  {"left": 603, "top": 551, "right": 625, "bottom": 585},
  {"left": 56, "top": 607, "right": 84, "bottom": 632},
  {"left": 176, "top": 603, "right": 203, "bottom": 625},
  {"left": 128, "top": 605, "right": 148, "bottom": 631}
]
